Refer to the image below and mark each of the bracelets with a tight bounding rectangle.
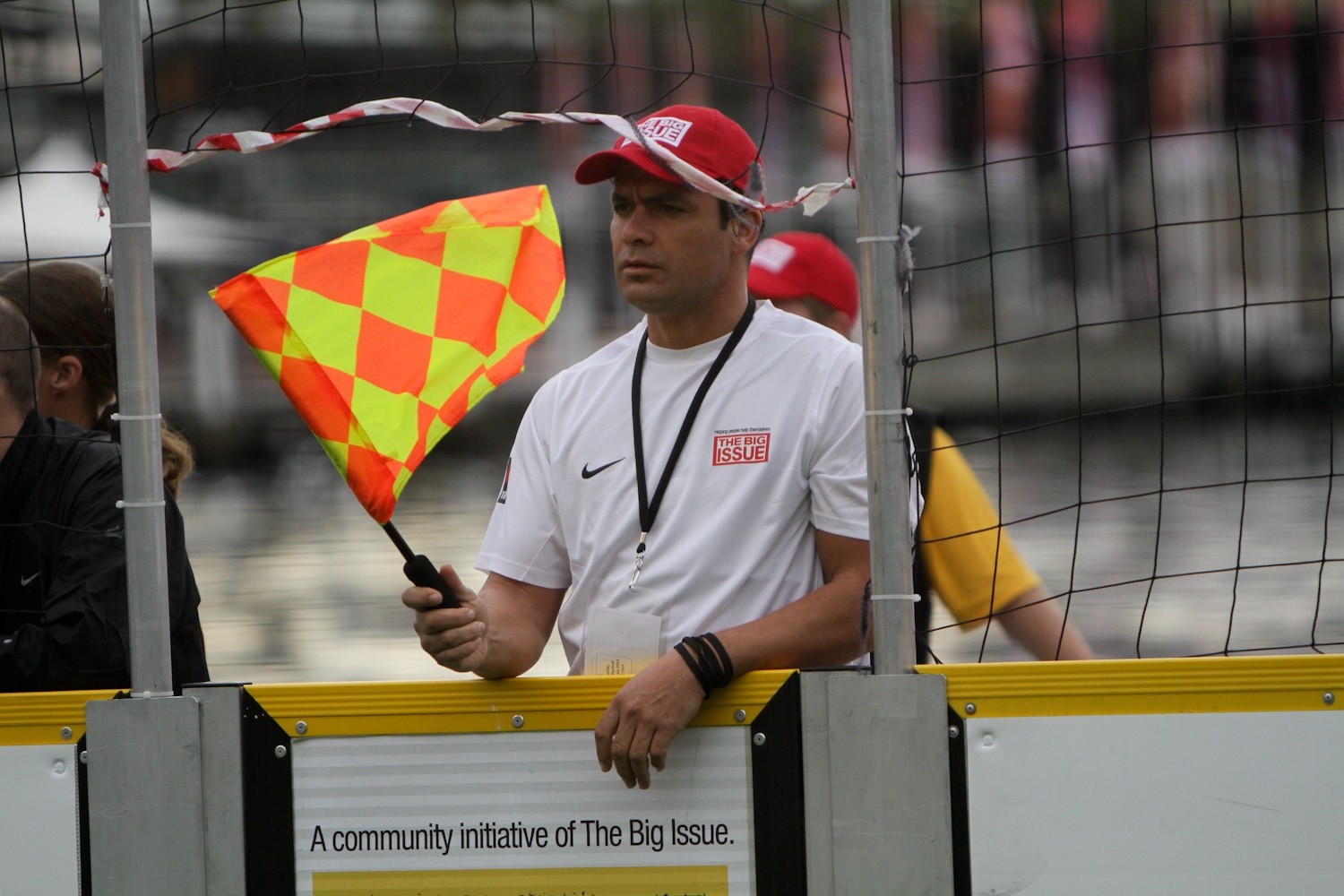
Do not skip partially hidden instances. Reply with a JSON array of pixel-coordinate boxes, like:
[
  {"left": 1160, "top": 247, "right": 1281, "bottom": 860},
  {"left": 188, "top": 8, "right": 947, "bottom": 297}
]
[{"left": 673, "top": 632, "right": 734, "bottom": 700}]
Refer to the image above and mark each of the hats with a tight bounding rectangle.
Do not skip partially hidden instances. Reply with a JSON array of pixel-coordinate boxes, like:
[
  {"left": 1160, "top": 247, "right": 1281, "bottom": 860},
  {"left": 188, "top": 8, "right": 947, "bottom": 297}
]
[
  {"left": 747, "top": 231, "right": 859, "bottom": 324},
  {"left": 574, "top": 104, "right": 766, "bottom": 219}
]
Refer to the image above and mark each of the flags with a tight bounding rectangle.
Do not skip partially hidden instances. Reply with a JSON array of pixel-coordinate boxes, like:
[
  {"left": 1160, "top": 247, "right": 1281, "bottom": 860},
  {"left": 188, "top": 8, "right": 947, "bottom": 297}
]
[{"left": 209, "top": 183, "right": 568, "bottom": 526}]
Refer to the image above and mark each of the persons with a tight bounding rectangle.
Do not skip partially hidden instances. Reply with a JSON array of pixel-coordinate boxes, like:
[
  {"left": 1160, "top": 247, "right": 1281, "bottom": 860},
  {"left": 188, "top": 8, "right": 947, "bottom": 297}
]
[
  {"left": 0, "top": 263, "right": 217, "bottom": 693},
  {"left": 400, "top": 106, "right": 924, "bottom": 789},
  {"left": 746, "top": 229, "right": 1097, "bottom": 663}
]
